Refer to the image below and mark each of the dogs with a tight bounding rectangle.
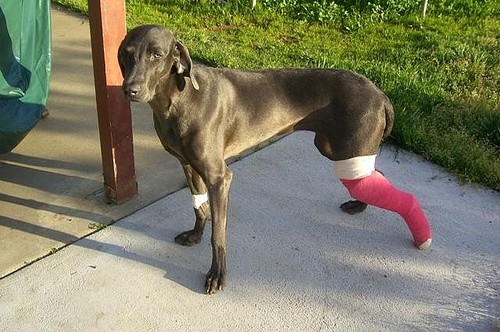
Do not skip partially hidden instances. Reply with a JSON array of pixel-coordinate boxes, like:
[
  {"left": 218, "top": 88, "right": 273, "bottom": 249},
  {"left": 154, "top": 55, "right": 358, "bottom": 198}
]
[{"left": 117, "top": 24, "right": 432, "bottom": 296}]
